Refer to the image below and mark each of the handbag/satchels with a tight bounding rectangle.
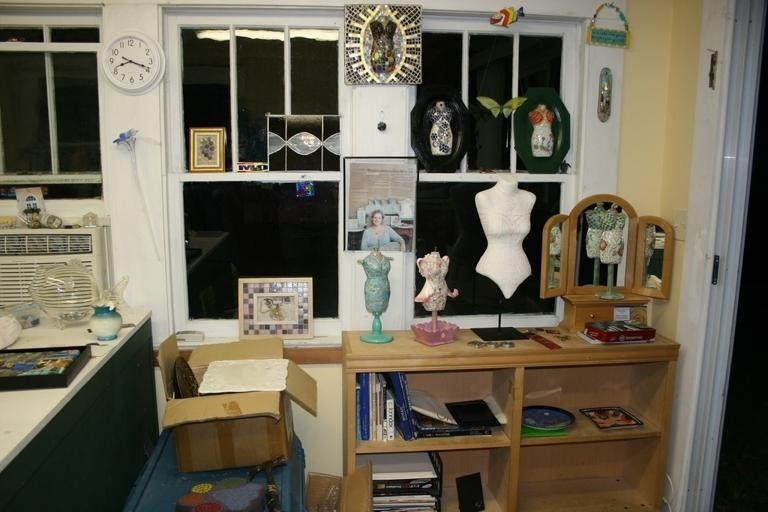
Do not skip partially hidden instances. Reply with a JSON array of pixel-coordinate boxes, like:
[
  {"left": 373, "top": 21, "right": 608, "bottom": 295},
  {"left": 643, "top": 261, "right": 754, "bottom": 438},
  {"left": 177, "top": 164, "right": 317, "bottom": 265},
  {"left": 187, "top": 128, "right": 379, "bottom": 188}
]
[{"left": 586, "top": 27, "right": 630, "bottom": 48}]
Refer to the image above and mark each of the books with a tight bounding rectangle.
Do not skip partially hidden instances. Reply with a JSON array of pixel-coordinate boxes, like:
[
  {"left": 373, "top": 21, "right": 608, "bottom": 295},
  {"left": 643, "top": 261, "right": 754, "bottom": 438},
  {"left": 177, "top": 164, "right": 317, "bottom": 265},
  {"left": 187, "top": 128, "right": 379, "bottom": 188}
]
[{"left": 354, "top": 371, "right": 506, "bottom": 511}]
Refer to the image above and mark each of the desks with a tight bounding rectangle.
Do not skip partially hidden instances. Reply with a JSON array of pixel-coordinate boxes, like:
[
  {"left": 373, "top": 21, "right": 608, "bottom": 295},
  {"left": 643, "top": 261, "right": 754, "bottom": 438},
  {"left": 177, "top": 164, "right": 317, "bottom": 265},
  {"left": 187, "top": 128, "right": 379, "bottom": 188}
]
[
  {"left": 0, "top": 308, "right": 159, "bottom": 512},
  {"left": 125, "top": 430, "right": 304, "bottom": 512}
]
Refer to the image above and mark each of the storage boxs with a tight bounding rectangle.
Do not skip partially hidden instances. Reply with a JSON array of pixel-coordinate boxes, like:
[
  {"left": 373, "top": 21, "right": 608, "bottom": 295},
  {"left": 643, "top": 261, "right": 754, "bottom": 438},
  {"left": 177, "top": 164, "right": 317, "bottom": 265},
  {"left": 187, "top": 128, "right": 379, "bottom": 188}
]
[
  {"left": 159, "top": 333, "right": 319, "bottom": 473},
  {"left": 306, "top": 460, "right": 373, "bottom": 512}
]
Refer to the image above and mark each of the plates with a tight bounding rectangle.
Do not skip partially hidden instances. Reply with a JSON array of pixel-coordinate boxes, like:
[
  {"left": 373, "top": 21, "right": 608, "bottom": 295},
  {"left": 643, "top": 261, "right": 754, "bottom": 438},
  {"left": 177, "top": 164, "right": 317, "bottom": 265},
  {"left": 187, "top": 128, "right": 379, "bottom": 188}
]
[{"left": 521, "top": 405, "right": 576, "bottom": 431}]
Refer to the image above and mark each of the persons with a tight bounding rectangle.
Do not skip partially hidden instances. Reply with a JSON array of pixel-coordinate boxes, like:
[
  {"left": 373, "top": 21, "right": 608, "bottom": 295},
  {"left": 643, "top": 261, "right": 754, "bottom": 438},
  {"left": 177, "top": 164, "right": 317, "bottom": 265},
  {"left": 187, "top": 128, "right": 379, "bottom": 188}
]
[
  {"left": 475, "top": 175, "right": 537, "bottom": 300},
  {"left": 419, "top": 251, "right": 450, "bottom": 312},
  {"left": 360, "top": 209, "right": 406, "bottom": 251},
  {"left": 424, "top": 101, "right": 452, "bottom": 157},
  {"left": 549, "top": 200, "right": 633, "bottom": 298},
  {"left": 369, "top": 15, "right": 398, "bottom": 74},
  {"left": 527, "top": 104, "right": 555, "bottom": 159},
  {"left": 362, "top": 248, "right": 391, "bottom": 314}
]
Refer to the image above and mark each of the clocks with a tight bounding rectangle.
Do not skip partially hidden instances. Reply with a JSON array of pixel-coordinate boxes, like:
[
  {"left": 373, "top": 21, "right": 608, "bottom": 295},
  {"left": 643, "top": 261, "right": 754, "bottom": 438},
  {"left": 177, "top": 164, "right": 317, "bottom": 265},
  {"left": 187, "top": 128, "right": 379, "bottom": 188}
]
[{"left": 100, "top": 30, "right": 165, "bottom": 96}]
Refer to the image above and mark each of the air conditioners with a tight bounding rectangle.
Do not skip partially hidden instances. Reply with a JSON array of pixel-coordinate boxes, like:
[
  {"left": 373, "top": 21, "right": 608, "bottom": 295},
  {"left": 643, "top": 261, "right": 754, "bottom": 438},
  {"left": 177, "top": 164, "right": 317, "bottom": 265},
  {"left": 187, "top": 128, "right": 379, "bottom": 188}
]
[{"left": 0, "top": 217, "right": 110, "bottom": 309}]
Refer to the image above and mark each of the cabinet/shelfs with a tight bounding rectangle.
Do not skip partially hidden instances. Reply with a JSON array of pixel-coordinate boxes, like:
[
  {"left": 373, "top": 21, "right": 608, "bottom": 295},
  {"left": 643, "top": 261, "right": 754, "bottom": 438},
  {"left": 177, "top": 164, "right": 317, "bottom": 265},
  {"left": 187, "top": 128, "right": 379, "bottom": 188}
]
[{"left": 342, "top": 328, "right": 680, "bottom": 512}]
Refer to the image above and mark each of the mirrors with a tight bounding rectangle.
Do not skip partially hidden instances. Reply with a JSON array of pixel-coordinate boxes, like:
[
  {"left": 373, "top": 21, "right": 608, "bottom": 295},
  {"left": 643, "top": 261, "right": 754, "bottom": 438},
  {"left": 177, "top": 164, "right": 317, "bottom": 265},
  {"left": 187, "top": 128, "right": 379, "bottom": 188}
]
[{"left": 540, "top": 194, "right": 674, "bottom": 332}]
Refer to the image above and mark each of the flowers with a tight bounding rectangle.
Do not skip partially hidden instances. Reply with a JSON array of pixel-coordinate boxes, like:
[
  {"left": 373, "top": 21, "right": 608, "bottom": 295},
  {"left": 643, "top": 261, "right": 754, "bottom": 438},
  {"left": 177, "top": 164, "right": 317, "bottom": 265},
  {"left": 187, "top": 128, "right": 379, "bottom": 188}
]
[{"left": 113, "top": 128, "right": 164, "bottom": 264}]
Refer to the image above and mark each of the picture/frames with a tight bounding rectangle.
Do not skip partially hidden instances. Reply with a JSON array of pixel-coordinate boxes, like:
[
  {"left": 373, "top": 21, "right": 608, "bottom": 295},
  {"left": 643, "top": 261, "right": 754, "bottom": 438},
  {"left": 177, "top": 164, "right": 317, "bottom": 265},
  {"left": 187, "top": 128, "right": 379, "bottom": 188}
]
[
  {"left": 190, "top": 127, "right": 227, "bottom": 172},
  {"left": 238, "top": 278, "right": 315, "bottom": 340},
  {"left": 342, "top": 156, "right": 419, "bottom": 253}
]
